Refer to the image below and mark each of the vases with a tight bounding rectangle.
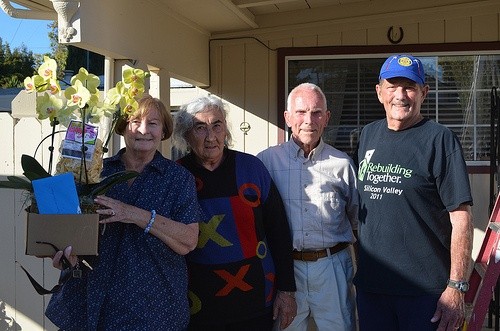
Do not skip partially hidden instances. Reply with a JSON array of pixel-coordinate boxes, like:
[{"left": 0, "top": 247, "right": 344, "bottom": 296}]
[{"left": 30, "top": 193, "right": 103, "bottom": 214}]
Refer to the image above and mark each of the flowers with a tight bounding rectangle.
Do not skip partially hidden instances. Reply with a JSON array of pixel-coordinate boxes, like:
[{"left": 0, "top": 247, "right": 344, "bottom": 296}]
[{"left": 21, "top": 55, "right": 151, "bottom": 197}]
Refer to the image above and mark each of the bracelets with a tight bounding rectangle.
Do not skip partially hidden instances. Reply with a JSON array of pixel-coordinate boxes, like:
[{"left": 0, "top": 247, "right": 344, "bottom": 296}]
[
  {"left": 281, "top": 292, "right": 296, "bottom": 299},
  {"left": 144, "top": 210, "right": 156, "bottom": 233}
]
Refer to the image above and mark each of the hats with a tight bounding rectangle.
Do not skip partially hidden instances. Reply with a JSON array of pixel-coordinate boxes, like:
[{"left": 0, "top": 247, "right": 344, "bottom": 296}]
[{"left": 379, "top": 54, "right": 425, "bottom": 86}]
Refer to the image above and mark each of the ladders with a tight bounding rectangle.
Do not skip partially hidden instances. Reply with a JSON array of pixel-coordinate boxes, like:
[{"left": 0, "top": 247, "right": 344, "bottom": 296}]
[{"left": 460, "top": 193, "right": 500, "bottom": 331}]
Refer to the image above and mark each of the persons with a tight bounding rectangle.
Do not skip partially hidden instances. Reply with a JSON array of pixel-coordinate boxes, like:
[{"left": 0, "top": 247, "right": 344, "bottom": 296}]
[
  {"left": 174, "top": 93, "right": 299, "bottom": 331},
  {"left": 352, "top": 55, "right": 474, "bottom": 331},
  {"left": 35, "top": 95, "right": 204, "bottom": 331},
  {"left": 255, "top": 83, "right": 360, "bottom": 331}
]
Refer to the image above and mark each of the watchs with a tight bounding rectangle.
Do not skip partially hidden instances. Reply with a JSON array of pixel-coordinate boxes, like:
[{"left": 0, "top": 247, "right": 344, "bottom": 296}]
[{"left": 447, "top": 279, "right": 470, "bottom": 293}]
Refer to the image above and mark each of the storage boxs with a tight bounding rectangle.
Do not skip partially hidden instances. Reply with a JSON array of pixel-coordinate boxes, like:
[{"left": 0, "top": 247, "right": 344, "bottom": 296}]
[{"left": 22, "top": 207, "right": 99, "bottom": 257}]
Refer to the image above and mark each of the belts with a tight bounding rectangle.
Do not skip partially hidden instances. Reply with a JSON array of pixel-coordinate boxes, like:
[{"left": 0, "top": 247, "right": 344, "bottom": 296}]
[{"left": 293, "top": 242, "right": 348, "bottom": 261}]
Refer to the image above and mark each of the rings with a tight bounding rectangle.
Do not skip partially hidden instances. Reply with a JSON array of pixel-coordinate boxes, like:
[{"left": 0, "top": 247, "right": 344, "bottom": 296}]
[
  {"left": 292, "top": 316, "right": 294, "bottom": 318},
  {"left": 455, "top": 326, "right": 459, "bottom": 329},
  {"left": 111, "top": 209, "right": 115, "bottom": 216}
]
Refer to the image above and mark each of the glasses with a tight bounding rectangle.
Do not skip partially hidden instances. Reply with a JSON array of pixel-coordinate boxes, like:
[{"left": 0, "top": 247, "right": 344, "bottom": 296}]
[{"left": 15, "top": 241, "right": 72, "bottom": 295}]
